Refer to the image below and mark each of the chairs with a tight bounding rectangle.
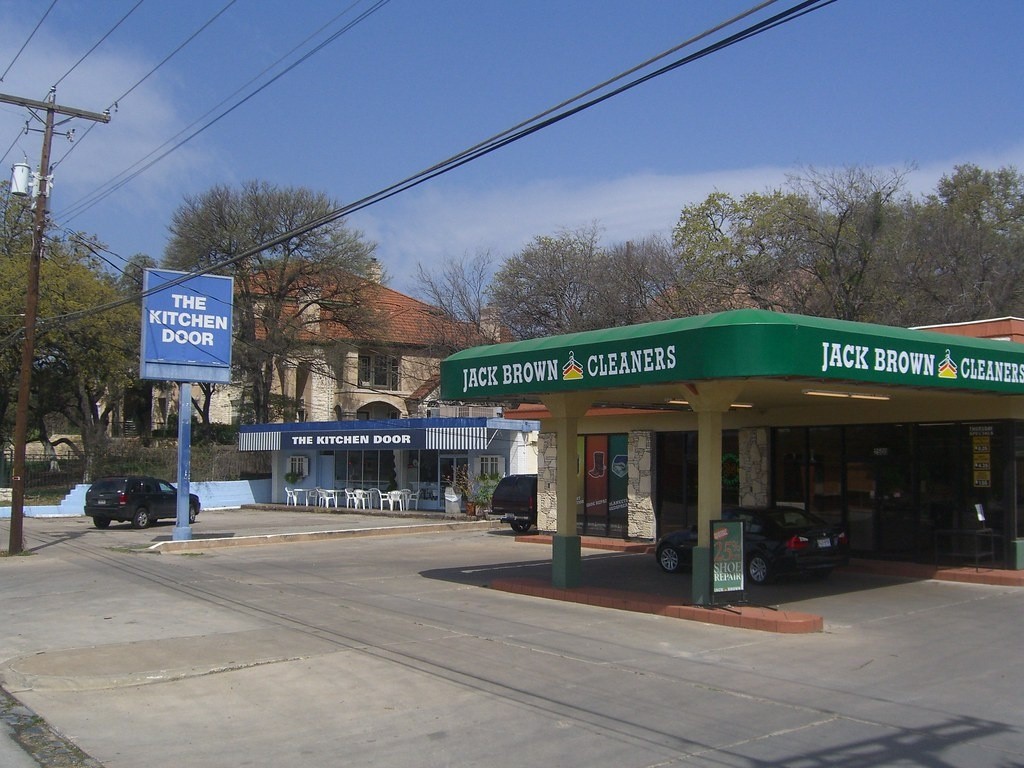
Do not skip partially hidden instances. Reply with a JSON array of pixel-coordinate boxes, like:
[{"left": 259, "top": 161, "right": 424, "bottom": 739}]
[
  {"left": 935, "top": 505, "right": 994, "bottom": 573},
  {"left": 308, "top": 486, "right": 322, "bottom": 506},
  {"left": 407, "top": 489, "right": 421, "bottom": 510},
  {"left": 316, "top": 489, "right": 336, "bottom": 508},
  {"left": 344, "top": 488, "right": 356, "bottom": 508},
  {"left": 285, "top": 487, "right": 301, "bottom": 506},
  {"left": 378, "top": 489, "right": 391, "bottom": 511},
  {"left": 389, "top": 490, "right": 403, "bottom": 511},
  {"left": 351, "top": 489, "right": 368, "bottom": 510},
  {"left": 401, "top": 489, "right": 411, "bottom": 511}
]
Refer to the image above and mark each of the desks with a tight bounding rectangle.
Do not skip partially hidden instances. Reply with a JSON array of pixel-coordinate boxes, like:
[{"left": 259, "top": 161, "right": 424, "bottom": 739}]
[
  {"left": 388, "top": 491, "right": 410, "bottom": 510},
  {"left": 294, "top": 488, "right": 313, "bottom": 506},
  {"left": 355, "top": 491, "right": 375, "bottom": 509},
  {"left": 322, "top": 490, "right": 346, "bottom": 508}
]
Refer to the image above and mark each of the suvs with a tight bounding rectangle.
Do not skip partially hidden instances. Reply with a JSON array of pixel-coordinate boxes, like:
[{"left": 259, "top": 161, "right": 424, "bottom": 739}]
[
  {"left": 488, "top": 474, "right": 538, "bottom": 533},
  {"left": 84, "top": 475, "right": 201, "bottom": 529}
]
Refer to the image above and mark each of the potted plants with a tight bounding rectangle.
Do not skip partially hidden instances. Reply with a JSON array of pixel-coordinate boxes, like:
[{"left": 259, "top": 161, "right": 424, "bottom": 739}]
[{"left": 441, "top": 463, "right": 501, "bottom": 515}]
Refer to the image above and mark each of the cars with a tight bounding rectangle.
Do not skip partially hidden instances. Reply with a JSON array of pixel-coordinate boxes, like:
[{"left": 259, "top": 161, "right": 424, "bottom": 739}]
[{"left": 655, "top": 506, "right": 849, "bottom": 586}]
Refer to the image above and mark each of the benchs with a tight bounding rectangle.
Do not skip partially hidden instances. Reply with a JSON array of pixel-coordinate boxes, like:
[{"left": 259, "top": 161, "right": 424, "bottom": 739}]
[{"left": 39, "top": 472, "right": 71, "bottom": 484}]
